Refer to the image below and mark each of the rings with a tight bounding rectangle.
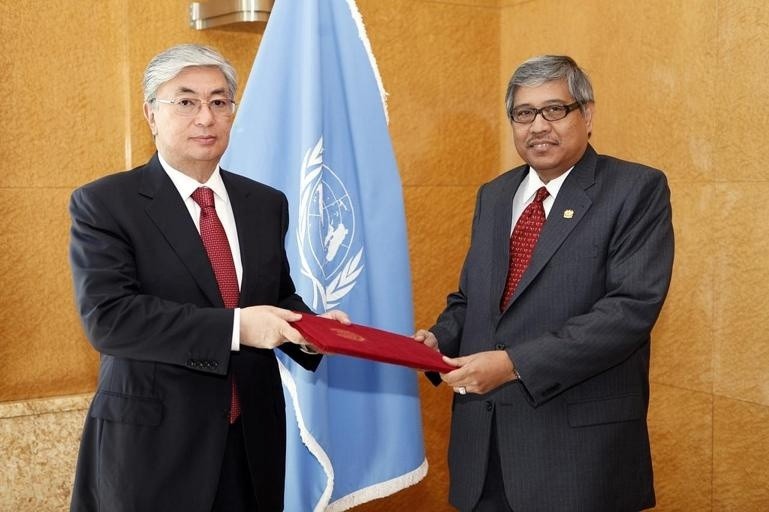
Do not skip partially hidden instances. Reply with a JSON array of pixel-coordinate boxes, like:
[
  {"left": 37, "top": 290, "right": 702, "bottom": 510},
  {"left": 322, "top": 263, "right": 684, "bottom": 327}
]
[{"left": 459, "top": 387, "right": 469, "bottom": 397}]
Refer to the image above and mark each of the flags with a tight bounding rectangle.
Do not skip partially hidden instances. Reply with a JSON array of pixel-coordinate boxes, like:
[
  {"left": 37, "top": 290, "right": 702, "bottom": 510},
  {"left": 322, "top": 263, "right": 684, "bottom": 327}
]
[{"left": 218, "top": 3, "right": 432, "bottom": 511}]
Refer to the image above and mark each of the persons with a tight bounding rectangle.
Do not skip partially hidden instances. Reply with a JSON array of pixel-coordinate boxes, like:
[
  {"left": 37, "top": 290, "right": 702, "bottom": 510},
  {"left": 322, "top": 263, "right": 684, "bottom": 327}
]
[
  {"left": 404, "top": 55, "right": 675, "bottom": 512},
  {"left": 68, "top": 52, "right": 354, "bottom": 512}
]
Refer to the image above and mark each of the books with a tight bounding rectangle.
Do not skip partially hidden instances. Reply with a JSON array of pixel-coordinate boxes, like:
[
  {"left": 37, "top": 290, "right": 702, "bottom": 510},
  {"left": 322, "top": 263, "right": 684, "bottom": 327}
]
[{"left": 285, "top": 311, "right": 462, "bottom": 376}]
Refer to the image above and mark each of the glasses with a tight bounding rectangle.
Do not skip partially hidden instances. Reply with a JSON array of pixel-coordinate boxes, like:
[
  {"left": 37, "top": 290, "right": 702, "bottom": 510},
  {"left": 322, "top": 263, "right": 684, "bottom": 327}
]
[
  {"left": 508, "top": 100, "right": 582, "bottom": 125},
  {"left": 149, "top": 96, "right": 238, "bottom": 117}
]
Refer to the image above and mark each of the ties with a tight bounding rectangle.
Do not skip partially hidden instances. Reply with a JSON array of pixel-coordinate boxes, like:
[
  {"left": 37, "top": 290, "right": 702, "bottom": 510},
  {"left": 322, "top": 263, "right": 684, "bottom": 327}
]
[
  {"left": 497, "top": 184, "right": 552, "bottom": 314},
  {"left": 188, "top": 186, "right": 245, "bottom": 427}
]
